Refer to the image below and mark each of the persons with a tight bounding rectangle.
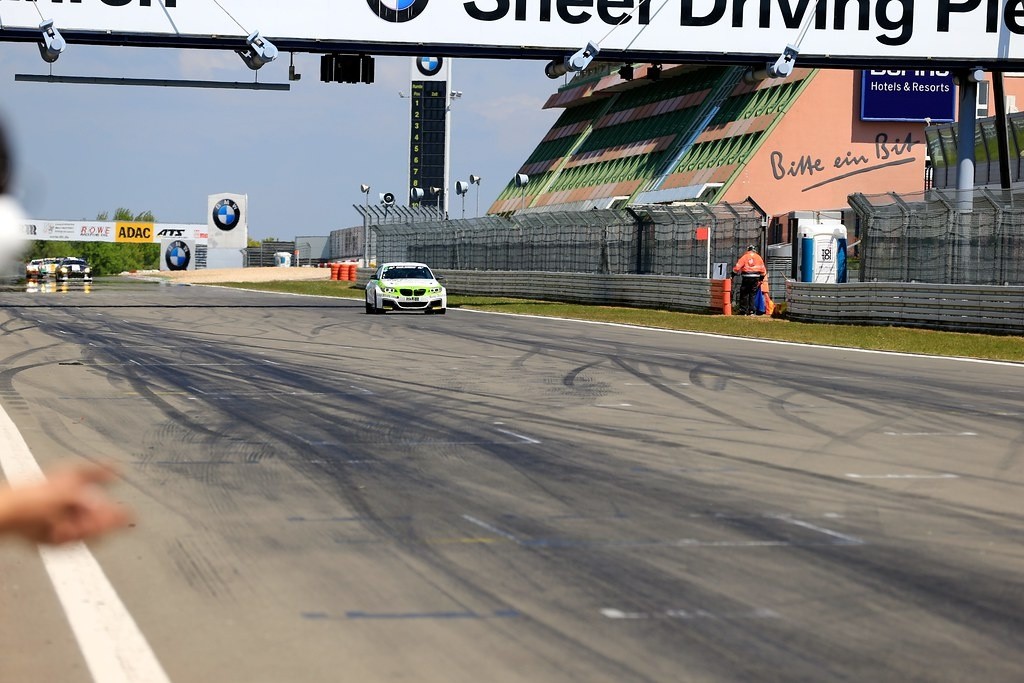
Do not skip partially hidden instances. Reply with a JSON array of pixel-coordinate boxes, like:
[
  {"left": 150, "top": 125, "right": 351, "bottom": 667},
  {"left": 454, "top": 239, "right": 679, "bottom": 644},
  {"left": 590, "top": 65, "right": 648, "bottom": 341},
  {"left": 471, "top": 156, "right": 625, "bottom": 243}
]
[
  {"left": 730, "top": 245, "right": 766, "bottom": 315},
  {"left": 0, "top": 459, "right": 135, "bottom": 547}
]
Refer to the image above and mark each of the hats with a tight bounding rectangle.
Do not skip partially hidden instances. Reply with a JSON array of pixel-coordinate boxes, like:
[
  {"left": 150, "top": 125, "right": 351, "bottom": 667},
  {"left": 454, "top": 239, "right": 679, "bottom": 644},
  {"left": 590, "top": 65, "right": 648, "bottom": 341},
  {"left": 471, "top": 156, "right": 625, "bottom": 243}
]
[{"left": 747, "top": 245, "right": 755, "bottom": 251}]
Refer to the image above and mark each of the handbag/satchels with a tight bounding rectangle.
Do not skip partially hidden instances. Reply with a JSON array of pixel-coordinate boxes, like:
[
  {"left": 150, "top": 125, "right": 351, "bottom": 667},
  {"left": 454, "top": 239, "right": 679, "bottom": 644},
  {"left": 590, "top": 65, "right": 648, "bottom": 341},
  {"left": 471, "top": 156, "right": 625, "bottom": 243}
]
[
  {"left": 763, "top": 292, "right": 776, "bottom": 315},
  {"left": 753, "top": 286, "right": 765, "bottom": 312}
]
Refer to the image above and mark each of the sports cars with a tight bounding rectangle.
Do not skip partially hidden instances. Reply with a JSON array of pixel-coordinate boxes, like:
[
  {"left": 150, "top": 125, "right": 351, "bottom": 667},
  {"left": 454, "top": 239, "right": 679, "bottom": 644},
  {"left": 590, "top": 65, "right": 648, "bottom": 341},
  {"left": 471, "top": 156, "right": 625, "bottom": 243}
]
[
  {"left": 25, "top": 256, "right": 92, "bottom": 282},
  {"left": 364, "top": 262, "right": 448, "bottom": 315}
]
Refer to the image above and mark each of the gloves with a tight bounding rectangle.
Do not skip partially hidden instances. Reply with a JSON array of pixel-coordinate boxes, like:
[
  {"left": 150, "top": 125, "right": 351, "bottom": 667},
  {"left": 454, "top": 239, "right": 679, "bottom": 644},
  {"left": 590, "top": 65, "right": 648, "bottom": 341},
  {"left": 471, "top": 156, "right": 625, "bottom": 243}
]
[
  {"left": 730, "top": 271, "right": 737, "bottom": 279},
  {"left": 759, "top": 275, "right": 765, "bottom": 282}
]
